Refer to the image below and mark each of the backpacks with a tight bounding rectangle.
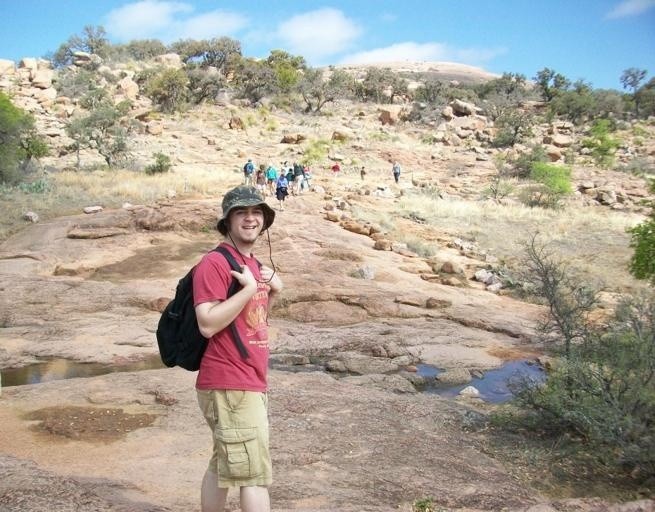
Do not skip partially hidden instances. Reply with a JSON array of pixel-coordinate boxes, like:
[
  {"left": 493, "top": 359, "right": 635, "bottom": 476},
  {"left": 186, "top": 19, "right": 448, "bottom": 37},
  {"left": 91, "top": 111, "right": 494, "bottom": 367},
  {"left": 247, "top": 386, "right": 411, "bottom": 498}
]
[{"left": 156, "top": 247, "right": 263, "bottom": 372}]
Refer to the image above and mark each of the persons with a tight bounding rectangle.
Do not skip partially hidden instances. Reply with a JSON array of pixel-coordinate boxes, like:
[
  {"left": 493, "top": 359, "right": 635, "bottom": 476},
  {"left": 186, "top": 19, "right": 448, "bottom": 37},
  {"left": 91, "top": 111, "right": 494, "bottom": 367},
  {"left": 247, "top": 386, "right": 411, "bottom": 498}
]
[
  {"left": 242, "top": 158, "right": 312, "bottom": 212},
  {"left": 391, "top": 160, "right": 402, "bottom": 184},
  {"left": 330, "top": 161, "right": 340, "bottom": 180},
  {"left": 190, "top": 185, "right": 285, "bottom": 512},
  {"left": 360, "top": 166, "right": 366, "bottom": 182}
]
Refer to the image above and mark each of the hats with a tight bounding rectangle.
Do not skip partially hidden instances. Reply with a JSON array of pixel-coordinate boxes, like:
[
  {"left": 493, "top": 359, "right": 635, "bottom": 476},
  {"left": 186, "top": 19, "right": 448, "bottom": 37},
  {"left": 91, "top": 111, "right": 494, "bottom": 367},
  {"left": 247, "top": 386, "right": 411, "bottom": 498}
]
[{"left": 217, "top": 185, "right": 275, "bottom": 235}]
